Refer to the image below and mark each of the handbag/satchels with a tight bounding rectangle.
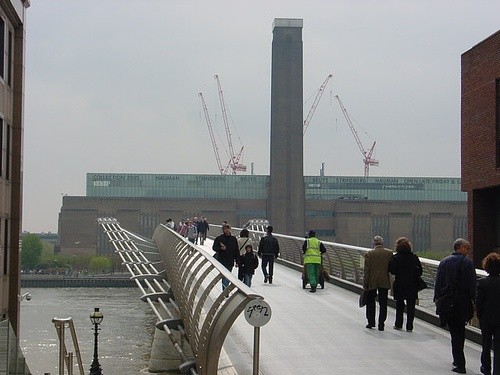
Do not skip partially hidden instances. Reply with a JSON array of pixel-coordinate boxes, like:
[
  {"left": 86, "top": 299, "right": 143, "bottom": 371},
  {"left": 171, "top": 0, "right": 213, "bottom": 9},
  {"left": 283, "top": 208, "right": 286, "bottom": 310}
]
[
  {"left": 238, "top": 250, "right": 240, "bottom": 255},
  {"left": 213, "top": 253, "right": 223, "bottom": 263},
  {"left": 418, "top": 277, "right": 427, "bottom": 291},
  {"left": 238, "top": 265, "right": 244, "bottom": 281},
  {"left": 360, "top": 291, "right": 367, "bottom": 307}
]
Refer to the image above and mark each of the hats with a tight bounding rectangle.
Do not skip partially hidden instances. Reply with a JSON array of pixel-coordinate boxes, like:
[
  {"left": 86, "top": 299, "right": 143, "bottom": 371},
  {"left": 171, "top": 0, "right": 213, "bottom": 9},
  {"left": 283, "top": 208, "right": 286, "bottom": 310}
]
[
  {"left": 372, "top": 236, "right": 384, "bottom": 244},
  {"left": 266, "top": 226, "right": 273, "bottom": 230}
]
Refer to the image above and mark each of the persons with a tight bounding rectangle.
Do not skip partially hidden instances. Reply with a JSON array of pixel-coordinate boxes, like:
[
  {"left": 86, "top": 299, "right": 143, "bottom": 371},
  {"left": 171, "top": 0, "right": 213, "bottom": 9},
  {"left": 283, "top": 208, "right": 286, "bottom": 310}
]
[
  {"left": 433, "top": 239, "right": 500, "bottom": 375},
  {"left": 302, "top": 229, "right": 327, "bottom": 293},
  {"left": 258, "top": 225, "right": 279, "bottom": 284},
  {"left": 359, "top": 236, "right": 426, "bottom": 332},
  {"left": 212, "top": 224, "right": 259, "bottom": 298},
  {"left": 164, "top": 216, "right": 209, "bottom": 245}
]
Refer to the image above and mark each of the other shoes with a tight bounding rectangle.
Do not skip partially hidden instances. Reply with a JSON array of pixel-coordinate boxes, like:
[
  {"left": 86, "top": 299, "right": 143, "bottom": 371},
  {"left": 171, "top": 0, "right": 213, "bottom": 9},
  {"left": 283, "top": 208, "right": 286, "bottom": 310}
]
[
  {"left": 264, "top": 276, "right": 268, "bottom": 283},
  {"left": 378, "top": 325, "right": 384, "bottom": 331},
  {"left": 481, "top": 365, "right": 491, "bottom": 375},
  {"left": 408, "top": 330, "right": 412, "bottom": 332},
  {"left": 394, "top": 326, "right": 401, "bottom": 330},
  {"left": 493, "top": 370, "right": 500, "bottom": 375},
  {"left": 269, "top": 276, "right": 272, "bottom": 283},
  {"left": 366, "top": 323, "right": 375, "bottom": 328},
  {"left": 453, "top": 367, "right": 466, "bottom": 374},
  {"left": 312, "top": 286, "right": 316, "bottom": 292}
]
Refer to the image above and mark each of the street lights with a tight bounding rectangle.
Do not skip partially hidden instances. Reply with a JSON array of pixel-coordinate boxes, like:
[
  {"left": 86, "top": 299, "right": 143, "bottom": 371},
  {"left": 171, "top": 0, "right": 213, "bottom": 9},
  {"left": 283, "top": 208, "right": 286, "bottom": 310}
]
[{"left": 88, "top": 308, "right": 104, "bottom": 375}]
